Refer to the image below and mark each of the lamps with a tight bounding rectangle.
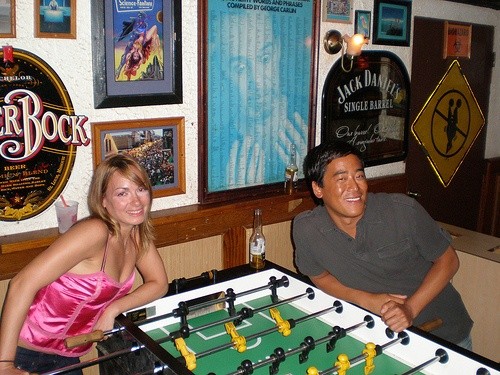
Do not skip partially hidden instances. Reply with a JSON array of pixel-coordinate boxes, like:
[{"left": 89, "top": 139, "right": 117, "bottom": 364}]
[{"left": 324, "top": 30, "right": 364, "bottom": 74}]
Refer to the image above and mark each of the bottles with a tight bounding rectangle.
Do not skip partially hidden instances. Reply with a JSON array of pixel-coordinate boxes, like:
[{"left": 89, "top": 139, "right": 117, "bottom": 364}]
[
  {"left": 249, "top": 209, "right": 266, "bottom": 269},
  {"left": 285, "top": 144, "right": 298, "bottom": 194}
]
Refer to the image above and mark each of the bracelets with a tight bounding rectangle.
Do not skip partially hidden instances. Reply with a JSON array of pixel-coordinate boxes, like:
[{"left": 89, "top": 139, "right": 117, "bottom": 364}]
[{"left": 0, "top": 360, "right": 15, "bottom": 362}]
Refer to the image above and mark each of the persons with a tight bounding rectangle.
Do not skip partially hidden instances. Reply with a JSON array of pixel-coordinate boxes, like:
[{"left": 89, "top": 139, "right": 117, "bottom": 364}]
[
  {"left": 292, "top": 138, "right": 474, "bottom": 351},
  {"left": 49, "top": 0, "right": 59, "bottom": 11},
  {"left": 0, "top": 152, "right": 168, "bottom": 375}
]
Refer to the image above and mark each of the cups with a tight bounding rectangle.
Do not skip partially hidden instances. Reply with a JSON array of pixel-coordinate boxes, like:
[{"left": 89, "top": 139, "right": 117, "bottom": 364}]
[{"left": 55, "top": 201, "right": 79, "bottom": 234}]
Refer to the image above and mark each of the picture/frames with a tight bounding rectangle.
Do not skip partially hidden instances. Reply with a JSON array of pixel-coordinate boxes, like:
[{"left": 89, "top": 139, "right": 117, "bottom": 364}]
[
  {"left": 322, "top": 0, "right": 354, "bottom": 25},
  {"left": 0, "top": 0, "right": 16, "bottom": 39},
  {"left": 372, "top": 0, "right": 412, "bottom": 47},
  {"left": 90, "top": 115, "right": 186, "bottom": 199},
  {"left": 197, "top": 0, "right": 322, "bottom": 205},
  {"left": 34, "top": 0, "right": 77, "bottom": 40},
  {"left": 90, "top": 0, "right": 183, "bottom": 109},
  {"left": 353, "top": 10, "right": 371, "bottom": 40}
]
